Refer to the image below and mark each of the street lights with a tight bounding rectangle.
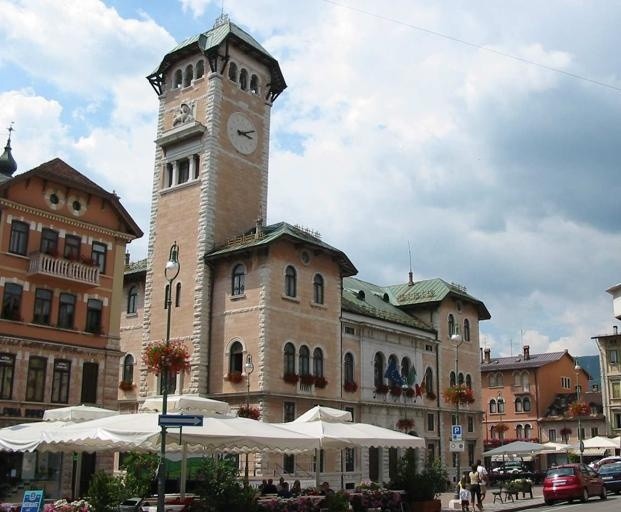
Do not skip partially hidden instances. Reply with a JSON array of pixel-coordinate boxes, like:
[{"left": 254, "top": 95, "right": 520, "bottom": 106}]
[
  {"left": 496, "top": 390, "right": 506, "bottom": 488},
  {"left": 243, "top": 353, "right": 254, "bottom": 488},
  {"left": 448, "top": 333, "right": 465, "bottom": 499},
  {"left": 573, "top": 360, "right": 583, "bottom": 464},
  {"left": 156, "top": 241, "right": 180, "bottom": 512}
]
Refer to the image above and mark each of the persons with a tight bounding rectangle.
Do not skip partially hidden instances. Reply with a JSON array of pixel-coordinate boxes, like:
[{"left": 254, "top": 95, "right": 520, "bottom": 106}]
[
  {"left": 476, "top": 459, "right": 490, "bottom": 509},
  {"left": 460, "top": 484, "right": 473, "bottom": 511},
  {"left": 470, "top": 464, "right": 486, "bottom": 511}
]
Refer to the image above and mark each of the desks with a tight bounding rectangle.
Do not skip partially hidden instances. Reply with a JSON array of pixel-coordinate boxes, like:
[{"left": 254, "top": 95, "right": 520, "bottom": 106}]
[{"left": 142, "top": 490, "right": 406, "bottom": 512}]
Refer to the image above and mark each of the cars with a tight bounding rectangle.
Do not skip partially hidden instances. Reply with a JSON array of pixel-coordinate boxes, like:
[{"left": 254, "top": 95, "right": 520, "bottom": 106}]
[
  {"left": 543, "top": 456, "right": 621, "bottom": 506},
  {"left": 493, "top": 462, "right": 532, "bottom": 474}
]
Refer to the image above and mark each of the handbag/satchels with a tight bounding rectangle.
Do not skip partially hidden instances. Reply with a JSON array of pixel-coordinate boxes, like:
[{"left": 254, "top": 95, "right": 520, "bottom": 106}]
[{"left": 477, "top": 476, "right": 482, "bottom": 483}]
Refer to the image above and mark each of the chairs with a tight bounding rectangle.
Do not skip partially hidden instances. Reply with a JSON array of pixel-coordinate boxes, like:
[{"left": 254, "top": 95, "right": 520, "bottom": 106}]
[{"left": 492, "top": 480, "right": 514, "bottom": 504}]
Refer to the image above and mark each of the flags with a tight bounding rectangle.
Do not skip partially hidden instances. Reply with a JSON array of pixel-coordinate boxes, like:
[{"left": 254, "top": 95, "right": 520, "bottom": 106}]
[
  {"left": 420, "top": 376, "right": 427, "bottom": 395},
  {"left": 385, "top": 359, "right": 406, "bottom": 385},
  {"left": 406, "top": 362, "right": 418, "bottom": 386}
]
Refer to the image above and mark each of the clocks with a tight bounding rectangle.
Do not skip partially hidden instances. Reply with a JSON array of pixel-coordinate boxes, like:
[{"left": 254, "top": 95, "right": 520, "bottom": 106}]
[{"left": 227, "top": 112, "right": 258, "bottom": 155}]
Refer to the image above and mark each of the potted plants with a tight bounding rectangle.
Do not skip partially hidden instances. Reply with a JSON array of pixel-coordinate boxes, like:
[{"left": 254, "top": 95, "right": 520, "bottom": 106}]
[{"left": 386, "top": 448, "right": 450, "bottom": 512}]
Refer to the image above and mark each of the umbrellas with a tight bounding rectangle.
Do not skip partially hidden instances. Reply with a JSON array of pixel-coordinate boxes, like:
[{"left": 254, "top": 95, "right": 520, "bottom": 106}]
[{"left": 483, "top": 435, "right": 621, "bottom": 456}]
